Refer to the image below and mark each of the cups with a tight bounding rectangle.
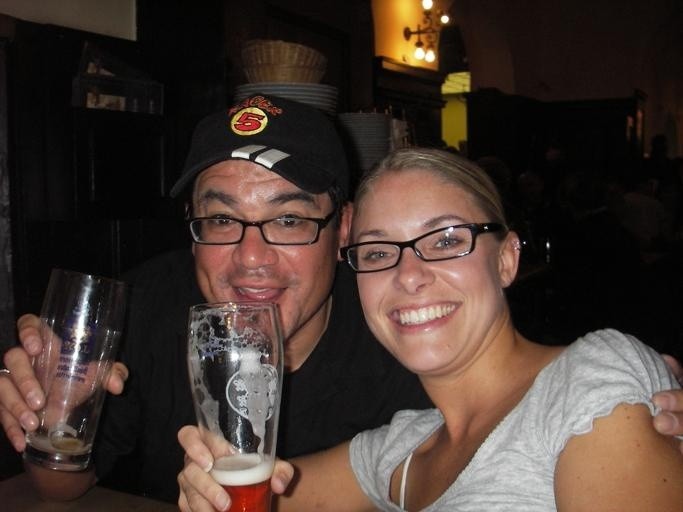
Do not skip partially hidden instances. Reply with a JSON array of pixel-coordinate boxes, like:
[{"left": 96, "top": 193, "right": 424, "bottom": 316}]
[
  {"left": 187, "top": 302, "right": 286, "bottom": 512},
  {"left": 21, "top": 268, "right": 128, "bottom": 474}
]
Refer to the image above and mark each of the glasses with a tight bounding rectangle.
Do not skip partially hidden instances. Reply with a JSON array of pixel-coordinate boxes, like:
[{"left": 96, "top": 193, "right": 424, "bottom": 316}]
[
  {"left": 182, "top": 205, "right": 336, "bottom": 245},
  {"left": 340, "top": 222, "right": 505, "bottom": 273}
]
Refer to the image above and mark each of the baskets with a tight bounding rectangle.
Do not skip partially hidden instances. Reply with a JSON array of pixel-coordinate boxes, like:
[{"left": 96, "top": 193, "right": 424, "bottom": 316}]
[{"left": 240, "top": 38, "right": 327, "bottom": 83}]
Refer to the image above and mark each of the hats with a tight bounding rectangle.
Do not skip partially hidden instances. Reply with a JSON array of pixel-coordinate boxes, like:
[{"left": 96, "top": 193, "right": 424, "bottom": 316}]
[{"left": 169, "top": 92, "right": 350, "bottom": 200}]
